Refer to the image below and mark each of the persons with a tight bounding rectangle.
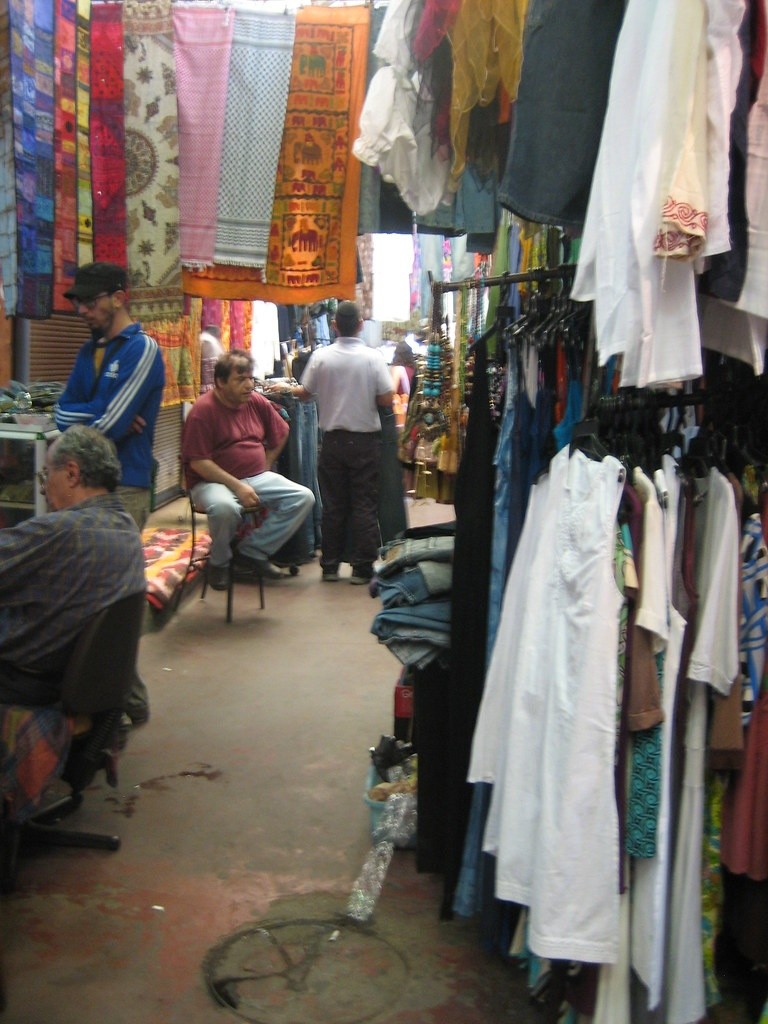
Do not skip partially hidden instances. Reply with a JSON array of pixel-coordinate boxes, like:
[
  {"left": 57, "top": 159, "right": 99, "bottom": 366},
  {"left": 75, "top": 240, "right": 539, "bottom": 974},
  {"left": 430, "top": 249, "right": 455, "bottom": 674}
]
[
  {"left": 264, "top": 301, "right": 416, "bottom": 584},
  {"left": 182, "top": 349, "right": 316, "bottom": 591},
  {"left": 54, "top": 260, "right": 166, "bottom": 724},
  {"left": 0, "top": 425, "right": 147, "bottom": 715}
]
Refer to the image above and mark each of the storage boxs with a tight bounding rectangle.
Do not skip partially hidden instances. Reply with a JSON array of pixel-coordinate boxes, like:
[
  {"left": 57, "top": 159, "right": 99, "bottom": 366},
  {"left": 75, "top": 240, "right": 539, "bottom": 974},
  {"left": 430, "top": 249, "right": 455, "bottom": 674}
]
[{"left": 362, "top": 766, "right": 416, "bottom": 851}]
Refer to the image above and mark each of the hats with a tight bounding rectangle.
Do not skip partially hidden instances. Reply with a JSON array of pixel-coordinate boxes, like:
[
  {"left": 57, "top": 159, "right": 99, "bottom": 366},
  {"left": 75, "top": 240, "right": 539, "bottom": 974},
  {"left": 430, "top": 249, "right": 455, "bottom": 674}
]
[{"left": 63, "top": 262, "right": 126, "bottom": 300}]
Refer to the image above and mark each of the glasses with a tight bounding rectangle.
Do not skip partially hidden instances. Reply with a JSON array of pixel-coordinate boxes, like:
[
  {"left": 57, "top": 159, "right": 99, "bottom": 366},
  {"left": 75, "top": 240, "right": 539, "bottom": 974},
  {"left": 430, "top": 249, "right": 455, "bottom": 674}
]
[
  {"left": 37, "top": 465, "right": 67, "bottom": 486},
  {"left": 71, "top": 291, "right": 114, "bottom": 310}
]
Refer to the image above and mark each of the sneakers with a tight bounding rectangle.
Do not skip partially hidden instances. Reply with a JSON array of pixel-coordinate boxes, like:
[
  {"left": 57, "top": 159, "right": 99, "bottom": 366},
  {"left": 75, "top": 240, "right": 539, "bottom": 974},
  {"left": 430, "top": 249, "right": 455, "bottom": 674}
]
[
  {"left": 323, "top": 564, "right": 338, "bottom": 580},
  {"left": 351, "top": 564, "right": 377, "bottom": 582},
  {"left": 230, "top": 547, "right": 285, "bottom": 578},
  {"left": 208, "top": 557, "right": 230, "bottom": 590}
]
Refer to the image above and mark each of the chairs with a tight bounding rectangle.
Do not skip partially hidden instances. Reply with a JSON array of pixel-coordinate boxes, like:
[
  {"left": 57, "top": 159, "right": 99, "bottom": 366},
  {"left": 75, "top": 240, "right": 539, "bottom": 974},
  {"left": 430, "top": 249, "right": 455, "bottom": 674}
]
[
  {"left": 173, "top": 463, "right": 265, "bottom": 626},
  {"left": 0, "top": 591, "right": 146, "bottom": 895}
]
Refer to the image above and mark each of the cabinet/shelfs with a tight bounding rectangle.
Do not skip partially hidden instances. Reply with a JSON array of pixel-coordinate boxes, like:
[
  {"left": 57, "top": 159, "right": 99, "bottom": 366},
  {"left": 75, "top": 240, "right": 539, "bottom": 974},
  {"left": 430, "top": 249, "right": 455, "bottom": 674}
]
[{"left": 0, "top": 428, "right": 63, "bottom": 518}]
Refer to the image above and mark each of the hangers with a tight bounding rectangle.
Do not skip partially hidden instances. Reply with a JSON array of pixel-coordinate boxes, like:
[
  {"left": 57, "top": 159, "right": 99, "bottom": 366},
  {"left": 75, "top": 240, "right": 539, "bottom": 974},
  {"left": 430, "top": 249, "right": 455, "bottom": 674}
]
[{"left": 461, "top": 263, "right": 768, "bottom": 526}]
